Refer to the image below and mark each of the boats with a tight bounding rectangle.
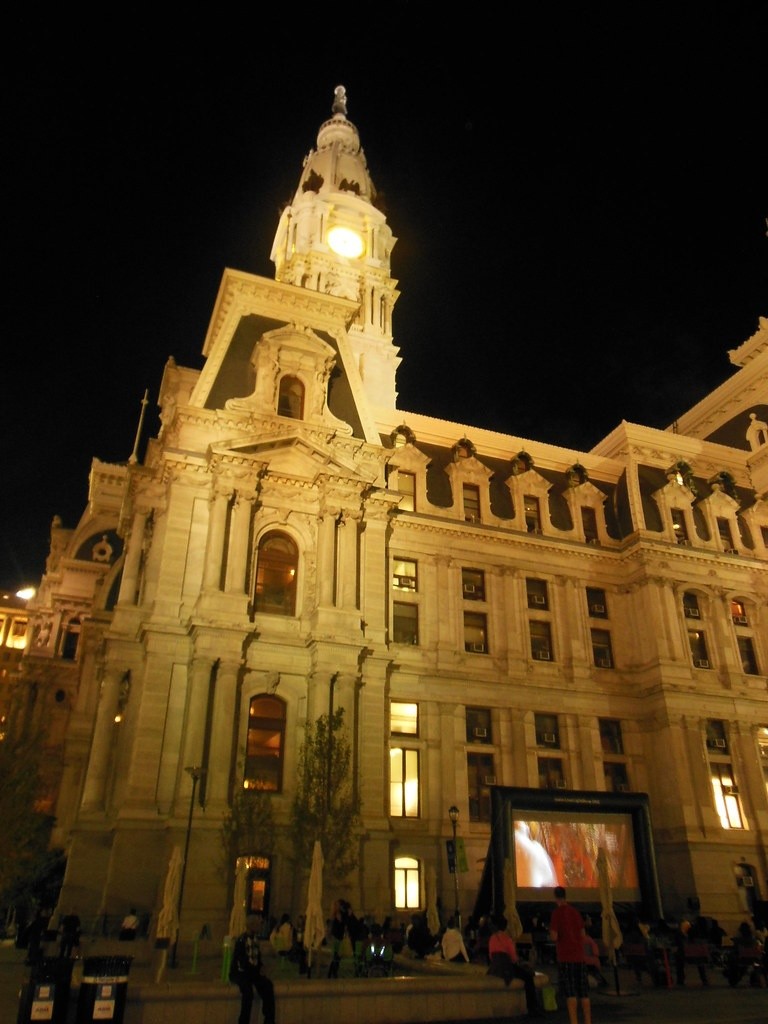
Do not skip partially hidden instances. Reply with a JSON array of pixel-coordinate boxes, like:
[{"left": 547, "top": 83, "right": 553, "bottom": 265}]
[{"left": 449, "top": 806, "right": 461, "bottom": 933}]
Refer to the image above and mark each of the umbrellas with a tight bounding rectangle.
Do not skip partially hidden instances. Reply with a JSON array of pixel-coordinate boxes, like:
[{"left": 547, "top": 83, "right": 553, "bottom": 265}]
[
  {"left": 596, "top": 848, "right": 623, "bottom": 996},
  {"left": 229, "top": 859, "right": 248, "bottom": 943},
  {"left": 156, "top": 846, "right": 185, "bottom": 950},
  {"left": 426, "top": 864, "right": 440, "bottom": 946},
  {"left": 502, "top": 859, "right": 523, "bottom": 940},
  {"left": 303, "top": 840, "right": 327, "bottom": 979}
]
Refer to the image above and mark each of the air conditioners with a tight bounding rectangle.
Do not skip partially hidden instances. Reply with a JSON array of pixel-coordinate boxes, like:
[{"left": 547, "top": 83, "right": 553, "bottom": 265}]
[
  {"left": 532, "top": 596, "right": 552, "bottom": 605},
  {"left": 680, "top": 540, "right": 692, "bottom": 546},
  {"left": 473, "top": 728, "right": 487, "bottom": 737},
  {"left": 470, "top": 518, "right": 483, "bottom": 525},
  {"left": 592, "top": 604, "right": 605, "bottom": 613},
  {"left": 687, "top": 609, "right": 699, "bottom": 617},
  {"left": 469, "top": 643, "right": 484, "bottom": 652},
  {"left": 538, "top": 651, "right": 550, "bottom": 659},
  {"left": 463, "top": 585, "right": 475, "bottom": 593},
  {"left": 696, "top": 660, "right": 709, "bottom": 668},
  {"left": 554, "top": 780, "right": 566, "bottom": 789},
  {"left": 590, "top": 539, "right": 601, "bottom": 546},
  {"left": 727, "top": 786, "right": 740, "bottom": 794},
  {"left": 399, "top": 578, "right": 413, "bottom": 586},
  {"left": 712, "top": 739, "right": 725, "bottom": 748},
  {"left": 734, "top": 617, "right": 747, "bottom": 624},
  {"left": 543, "top": 734, "right": 557, "bottom": 742},
  {"left": 532, "top": 528, "right": 543, "bottom": 535},
  {"left": 727, "top": 549, "right": 738, "bottom": 554},
  {"left": 485, "top": 776, "right": 495, "bottom": 785},
  {"left": 740, "top": 876, "right": 753, "bottom": 890}
]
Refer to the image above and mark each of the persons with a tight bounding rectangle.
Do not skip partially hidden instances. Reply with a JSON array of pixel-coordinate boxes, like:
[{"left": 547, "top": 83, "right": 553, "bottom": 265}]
[
  {"left": 549, "top": 886, "right": 591, "bottom": 1024},
  {"left": 22, "top": 903, "right": 142, "bottom": 960},
  {"left": 675, "top": 919, "right": 768, "bottom": 991},
  {"left": 227, "top": 898, "right": 544, "bottom": 1024},
  {"left": 331, "top": 85, "right": 350, "bottom": 116},
  {"left": 311, "top": 371, "right": 326, "bottom": 416},
  {"left": 256, "top": 358, "right": 281, "bottom": 412}
]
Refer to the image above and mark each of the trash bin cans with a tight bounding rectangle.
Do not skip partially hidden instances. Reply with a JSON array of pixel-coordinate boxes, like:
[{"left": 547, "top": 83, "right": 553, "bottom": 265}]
[
  {"left": 78, "top": 955, "right": 135, "bottom": 1024},
  {"left": 16, "top": 955, "right": 80, "bottom": 1024}
]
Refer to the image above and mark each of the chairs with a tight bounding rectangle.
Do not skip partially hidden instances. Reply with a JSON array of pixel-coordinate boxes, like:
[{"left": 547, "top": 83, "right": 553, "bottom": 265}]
[
  {"left": 623, "top": 942, "right": 762, "bottom": 987},
  {"left": 516, "top": 933, "right": 533, "bottom": 961}
]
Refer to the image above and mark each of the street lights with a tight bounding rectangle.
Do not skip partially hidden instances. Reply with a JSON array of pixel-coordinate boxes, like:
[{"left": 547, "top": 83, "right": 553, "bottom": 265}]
[{"left": 167, "top": 767, "right": 210, "bottom": 969}]
